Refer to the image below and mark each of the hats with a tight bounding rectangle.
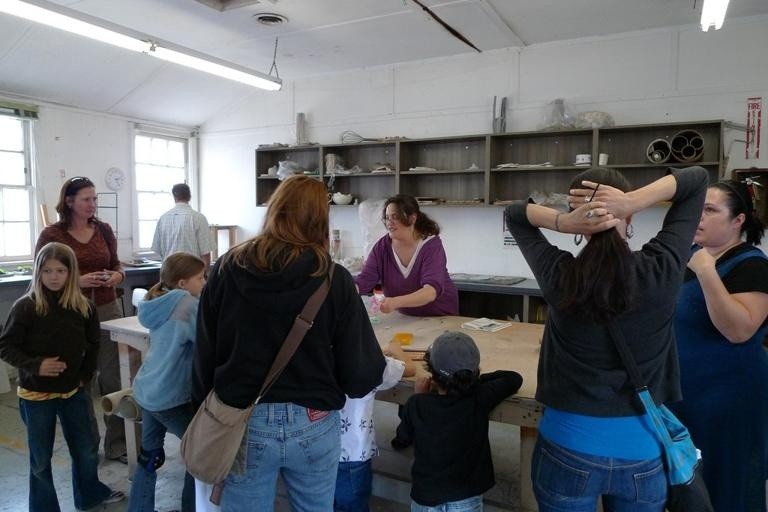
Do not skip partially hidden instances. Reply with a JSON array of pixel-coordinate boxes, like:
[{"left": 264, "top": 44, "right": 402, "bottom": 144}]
[{"left": 428, "top": 331, "right": 479, "bottom": 381}]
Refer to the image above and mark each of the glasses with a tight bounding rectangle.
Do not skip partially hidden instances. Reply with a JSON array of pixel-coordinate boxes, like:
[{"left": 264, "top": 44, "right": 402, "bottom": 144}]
[{"left": 68, "top": 177, "right": 90, "bottom": 185}]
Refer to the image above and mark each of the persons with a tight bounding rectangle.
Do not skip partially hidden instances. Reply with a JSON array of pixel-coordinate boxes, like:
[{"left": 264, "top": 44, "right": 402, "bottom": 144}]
[
  {"left": 505, "top": 165, "right": 710, "bottom": 511},
  {"left": 0, "top": 240, "right": 125, "bottom": 511},
  {"left": 389, "top": 330, "right": 524, "bottom": 512},
  {"left": 34, "top": 175, "right": 130, "bottom": 466},
  {"left": 125, "top": 253, "right": 208, "bottom": 511},
  {"left": 330, "top": 338, "right": 417, "bottom": 512},
  {"left": 673, "top": 173, "right": 768, "bottom": 511},
  {"left": 191, "top": 173, "right": 388, "bottom": 512},
  {"left": 148, "top": 180, "right": 215, "bottom": 272},
  {"left": 354, "top": 194, "right": 459, "bottom": 451}
]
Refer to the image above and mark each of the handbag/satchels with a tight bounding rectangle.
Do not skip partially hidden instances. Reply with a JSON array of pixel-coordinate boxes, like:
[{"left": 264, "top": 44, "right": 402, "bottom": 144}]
[
  {"left": 179, "top": 390, "right": 256, "bottom": 483},
  {"left": 659, "top": 404, "right": 716, "bottom": 512}
]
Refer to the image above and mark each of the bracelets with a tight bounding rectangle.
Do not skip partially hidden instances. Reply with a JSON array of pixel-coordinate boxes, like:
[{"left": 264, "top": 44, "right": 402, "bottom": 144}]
[{"left": 555, "top": 212, "right": 568, "bottom": 232}]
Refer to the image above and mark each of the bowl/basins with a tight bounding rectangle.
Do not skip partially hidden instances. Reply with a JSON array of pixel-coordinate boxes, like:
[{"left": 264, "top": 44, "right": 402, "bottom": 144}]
[{"left": 333, "top": 195, "right": 353, "bottom": 205}]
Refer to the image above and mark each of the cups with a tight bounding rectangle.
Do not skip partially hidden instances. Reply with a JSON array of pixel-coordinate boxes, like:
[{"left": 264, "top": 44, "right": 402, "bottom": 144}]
[{"left": 575, "top": 154, "right": 592, "bottom": 167}]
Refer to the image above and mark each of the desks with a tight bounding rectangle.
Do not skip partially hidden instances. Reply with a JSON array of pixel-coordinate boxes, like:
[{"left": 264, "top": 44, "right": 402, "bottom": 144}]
[
  {"left": 0, "top": 260, "right": 216, "bottom": 394},
  {"left": 449, "top": 273, "right": 550, "bottom": 325},
  {"left": 100, "top": 293, "right": 546, "bottom": 512}
]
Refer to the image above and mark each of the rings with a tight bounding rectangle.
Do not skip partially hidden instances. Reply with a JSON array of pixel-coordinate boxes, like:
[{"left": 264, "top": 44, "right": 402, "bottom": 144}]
[
  {"left": 584, "top": 196, "right": 595, "bottom": 202},
  {"left": 92, "top": 275, "right": 96, "bottom": 280},
  {"left": 585, "top": 209, "right": 594, "bottom": 217}
]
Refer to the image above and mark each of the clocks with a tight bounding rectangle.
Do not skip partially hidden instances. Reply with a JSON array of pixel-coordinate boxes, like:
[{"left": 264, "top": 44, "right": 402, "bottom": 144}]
[{"left": 104, "top": 167, "right": 126, "bottom": 191}]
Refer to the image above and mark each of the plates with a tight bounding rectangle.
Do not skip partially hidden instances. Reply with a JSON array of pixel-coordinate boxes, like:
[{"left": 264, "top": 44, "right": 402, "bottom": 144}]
[
  {"left": 120, "top": 258, "right": 154, "bottom": 266},
  {"left": 0, "top": 267, "right": 33, "bottom": 277}
]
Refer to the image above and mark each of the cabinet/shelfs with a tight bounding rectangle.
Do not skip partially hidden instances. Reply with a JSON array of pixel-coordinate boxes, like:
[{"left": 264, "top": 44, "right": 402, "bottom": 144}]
[{"left": 255, "top": 119, "right": 724, "bottom": 207}]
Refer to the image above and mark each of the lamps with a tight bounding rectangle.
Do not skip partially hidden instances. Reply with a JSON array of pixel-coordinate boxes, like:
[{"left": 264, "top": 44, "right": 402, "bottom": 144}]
[
  {"left": 0, "top": 0, "right": 283, "bottom": 92},
  {"left": 700, "top": 0, "right": 729, "bottom": 32}
]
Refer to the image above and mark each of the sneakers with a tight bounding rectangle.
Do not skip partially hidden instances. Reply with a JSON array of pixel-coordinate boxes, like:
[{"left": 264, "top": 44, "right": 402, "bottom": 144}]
[
  {"left": 391, "top": 435, "right": 412, "bottom": 451},
  {"left": 101, "top": 491, "right": 125, "bottom": 506}
]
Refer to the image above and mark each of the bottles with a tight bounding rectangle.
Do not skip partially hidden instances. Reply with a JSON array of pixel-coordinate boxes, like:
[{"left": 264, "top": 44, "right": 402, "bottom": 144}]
[
  {"left": 295, "top": 112, "right": 305, "bottom": 146},
  {"left": 368, "top": 284, "right": 387, "bottom": 323},
  {"left": 329, "top": 230, "right": 342, "bottom": 265}
]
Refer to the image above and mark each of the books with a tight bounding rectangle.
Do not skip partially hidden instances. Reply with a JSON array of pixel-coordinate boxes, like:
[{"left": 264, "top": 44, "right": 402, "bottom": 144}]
[{"left": 459, "top": 316, "right": 511, "bottom": 334}]
